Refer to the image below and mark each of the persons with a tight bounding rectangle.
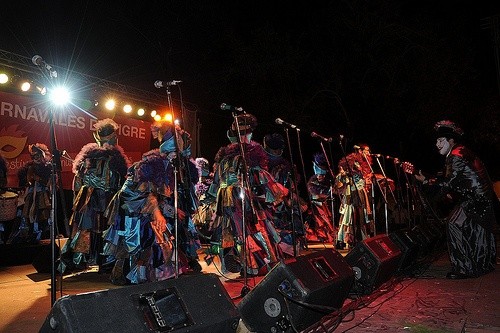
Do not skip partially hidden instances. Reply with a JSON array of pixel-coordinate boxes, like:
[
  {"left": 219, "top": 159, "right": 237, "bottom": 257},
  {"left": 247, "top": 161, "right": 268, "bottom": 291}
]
[
  {"left": 56, "top": 118, "right": 127, "bottom": 274},
  {"left": 191, "top": 114, "right": 307, "bottom": 276},
  {"left": 414, "top": 120, "right": 500, "bottom": 280},
  {"left": 101, "top": 122, "right": 202, "bottom": 285},
  {"left": 18, "top": 144, "right": 59, "bottom": 242},
  {"left": 263, "top": 134, "right": 334, "bottom": 258},
  {"left": 334, "top": 143, "right": 395, "bottom": 249}
]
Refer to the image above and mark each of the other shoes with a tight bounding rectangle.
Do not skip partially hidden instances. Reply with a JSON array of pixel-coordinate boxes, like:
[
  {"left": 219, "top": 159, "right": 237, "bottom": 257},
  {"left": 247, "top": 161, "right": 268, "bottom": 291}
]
[
  {"left": 446, "top": 272, "right": 464, "bottom": 279},
  {"left": 110, "top": 270, "right": 127, "bottom": 286},
  {"left": 337, "top": 242, "right": 343, "bottom": 249}
]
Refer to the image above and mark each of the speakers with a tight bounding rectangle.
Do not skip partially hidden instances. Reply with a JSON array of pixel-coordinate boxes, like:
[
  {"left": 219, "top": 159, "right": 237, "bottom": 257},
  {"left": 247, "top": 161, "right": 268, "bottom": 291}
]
[{"left": 38, "top": 223, "right": 438, "bottom": 333}]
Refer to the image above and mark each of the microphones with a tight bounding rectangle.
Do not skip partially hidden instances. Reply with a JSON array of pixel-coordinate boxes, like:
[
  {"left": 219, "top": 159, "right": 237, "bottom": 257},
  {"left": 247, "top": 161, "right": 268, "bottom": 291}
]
[
  {"left": 154, "top": 80, "right": 181, "bottom": 88},
  {"left": 275, "top": 118, "right": 300, "bottom": 131},
  {"left": 386, "top": 156, "right": 398, "bottom": 160},
  {"left": 338, "top": 135, "right": 363, "bottom": 150},
  {"left": 32, "top": 55, "right": 57, "bottom": 78},
  {"left": 369, "top": 154, "right": 381, "bottom": 157},
  {"left": 311, "top": 132, "right": 328, "bottom": 141},
  {"left": 220, "top": 103, "right": 243, "bottom": 112}
]
